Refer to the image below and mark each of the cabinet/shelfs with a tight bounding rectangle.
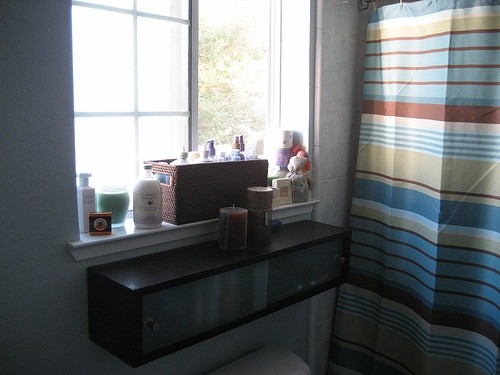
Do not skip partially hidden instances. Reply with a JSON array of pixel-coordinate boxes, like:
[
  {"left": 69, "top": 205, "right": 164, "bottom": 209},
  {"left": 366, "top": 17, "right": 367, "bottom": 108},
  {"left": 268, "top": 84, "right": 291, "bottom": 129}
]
[{"left": 86, "top": 218, "right": 354, "bottom": 369}]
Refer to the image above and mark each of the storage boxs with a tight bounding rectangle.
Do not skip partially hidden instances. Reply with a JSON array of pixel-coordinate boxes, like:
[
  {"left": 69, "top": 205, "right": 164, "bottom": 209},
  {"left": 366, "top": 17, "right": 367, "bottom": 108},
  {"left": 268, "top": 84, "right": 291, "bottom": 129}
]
[{"left": 141, "top": 155, "right": 269, "bottom": 227}]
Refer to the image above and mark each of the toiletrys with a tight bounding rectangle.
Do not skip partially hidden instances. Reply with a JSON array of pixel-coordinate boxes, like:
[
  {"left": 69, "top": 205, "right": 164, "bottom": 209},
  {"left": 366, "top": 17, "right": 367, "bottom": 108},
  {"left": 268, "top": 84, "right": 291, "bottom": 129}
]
[
  {"left": 76, "top": 171, "right": 97, "bottom": 234},
  {"left": 154, "top": 134, "right": 259, "bottom": 184},
  {"left": 131, "top": 162, "right": 165, "bottom": 230}
]
